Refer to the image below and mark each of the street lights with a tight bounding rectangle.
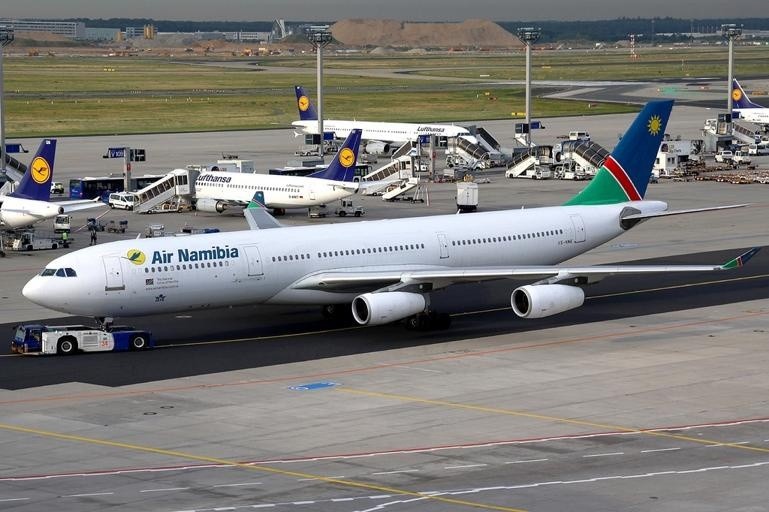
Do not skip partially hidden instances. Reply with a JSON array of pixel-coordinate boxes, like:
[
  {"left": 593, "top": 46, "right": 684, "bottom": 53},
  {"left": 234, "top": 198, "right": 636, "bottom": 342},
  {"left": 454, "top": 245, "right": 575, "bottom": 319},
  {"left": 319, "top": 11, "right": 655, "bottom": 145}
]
[
  {"left": 718, "top": 22, "right": 745, "bottom": 112},
  {"left": 515, "top": 26, "right": 543, "bottom": 156},
  {"left": 304, "top": 29, "right": 334, "bottom": 157}
]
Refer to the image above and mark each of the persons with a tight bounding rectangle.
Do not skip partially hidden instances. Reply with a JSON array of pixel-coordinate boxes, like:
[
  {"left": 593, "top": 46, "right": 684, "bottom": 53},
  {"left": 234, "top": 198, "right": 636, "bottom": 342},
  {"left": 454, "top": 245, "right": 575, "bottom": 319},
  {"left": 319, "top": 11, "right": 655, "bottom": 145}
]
[
  {"left": 90, "top": 227, "right": 97, "bottom": 245},
  {"left": 61, "top": 230, "right": 68, "bottom": 248}
]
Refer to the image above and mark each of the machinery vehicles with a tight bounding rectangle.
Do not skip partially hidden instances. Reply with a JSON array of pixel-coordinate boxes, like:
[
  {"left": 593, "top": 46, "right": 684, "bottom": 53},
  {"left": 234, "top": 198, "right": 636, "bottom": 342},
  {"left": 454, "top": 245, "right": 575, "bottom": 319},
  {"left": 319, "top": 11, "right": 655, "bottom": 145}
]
[{"left": 8, "top": 323, "right": 155, "bottom": 353}]
